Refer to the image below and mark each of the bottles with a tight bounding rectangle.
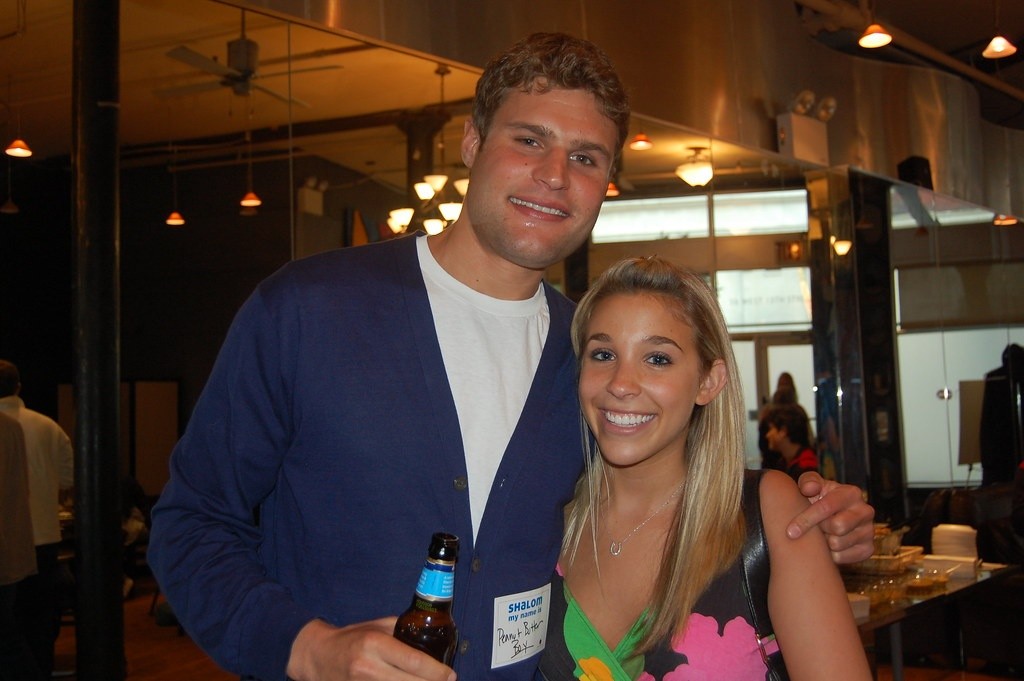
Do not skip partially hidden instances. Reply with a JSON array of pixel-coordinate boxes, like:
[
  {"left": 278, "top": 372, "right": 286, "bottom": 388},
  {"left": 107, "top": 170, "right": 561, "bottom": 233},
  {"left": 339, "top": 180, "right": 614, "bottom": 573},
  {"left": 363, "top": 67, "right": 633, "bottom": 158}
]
[{"left": 393, "top": 532, "right": 460, "bottom": 672}]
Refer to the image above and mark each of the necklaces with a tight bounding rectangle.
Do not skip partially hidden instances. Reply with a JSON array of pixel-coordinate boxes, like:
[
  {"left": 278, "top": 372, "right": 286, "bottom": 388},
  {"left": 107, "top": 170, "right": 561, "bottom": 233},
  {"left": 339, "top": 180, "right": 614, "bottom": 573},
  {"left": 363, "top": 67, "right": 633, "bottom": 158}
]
[{"left": 600, "top": 481, "right": 685, "bottom": 556}]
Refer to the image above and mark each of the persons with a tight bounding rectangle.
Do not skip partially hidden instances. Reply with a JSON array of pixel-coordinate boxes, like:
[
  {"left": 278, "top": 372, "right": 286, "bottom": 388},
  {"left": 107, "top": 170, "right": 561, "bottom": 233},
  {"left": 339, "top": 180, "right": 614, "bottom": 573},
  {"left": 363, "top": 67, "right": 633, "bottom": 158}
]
[
  {"left": 532, "top": 255, "right": 874, "bottom": 681},
  {"left": 981, "top": 345, "right": 1024, "bottom": 486},
  {"left": 0, "top": 360, "right": 75, "bottom": 681},
  {"left": 766, "top": 404, "right": 821, "bottom": 482},
  {"left": 758, "top": 372, "right": 818, "bottom": 474},
  {"left": 145, "top": 30, "right": 873, "bottom": 681},
  {"left": 0, "top": 410, "right": 39, "bottom": 679}
]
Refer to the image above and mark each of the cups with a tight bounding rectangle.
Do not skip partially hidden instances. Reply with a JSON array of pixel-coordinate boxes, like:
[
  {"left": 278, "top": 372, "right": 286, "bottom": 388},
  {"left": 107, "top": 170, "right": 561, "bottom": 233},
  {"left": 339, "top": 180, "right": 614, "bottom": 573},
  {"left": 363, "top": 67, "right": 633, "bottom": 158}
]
[{"left": 848, "top": 534, "right": 904, "bottom": 604}]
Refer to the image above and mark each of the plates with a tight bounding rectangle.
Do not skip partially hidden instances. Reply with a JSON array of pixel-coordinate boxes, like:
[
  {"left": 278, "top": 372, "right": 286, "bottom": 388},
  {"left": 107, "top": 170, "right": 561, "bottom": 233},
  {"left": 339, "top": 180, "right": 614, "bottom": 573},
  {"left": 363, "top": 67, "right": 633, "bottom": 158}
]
[{"left": 933, "top": 524, "right": 977, "bottom": 556}]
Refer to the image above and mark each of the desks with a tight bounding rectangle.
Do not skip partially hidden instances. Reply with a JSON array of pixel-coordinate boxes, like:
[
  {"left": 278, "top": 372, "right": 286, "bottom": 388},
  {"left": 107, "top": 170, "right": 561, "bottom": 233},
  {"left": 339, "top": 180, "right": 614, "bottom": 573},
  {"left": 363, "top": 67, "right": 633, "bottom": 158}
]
[{"left": 843, "top": 554, "right": 1023, "bottom": 681}]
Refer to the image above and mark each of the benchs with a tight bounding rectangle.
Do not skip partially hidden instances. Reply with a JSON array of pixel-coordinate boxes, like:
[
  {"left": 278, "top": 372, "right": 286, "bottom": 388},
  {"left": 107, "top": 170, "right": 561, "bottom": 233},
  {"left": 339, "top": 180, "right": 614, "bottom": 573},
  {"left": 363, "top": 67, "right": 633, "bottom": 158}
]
[{"left": 876, "top": 482, "right": 1023, "bottom": 677}]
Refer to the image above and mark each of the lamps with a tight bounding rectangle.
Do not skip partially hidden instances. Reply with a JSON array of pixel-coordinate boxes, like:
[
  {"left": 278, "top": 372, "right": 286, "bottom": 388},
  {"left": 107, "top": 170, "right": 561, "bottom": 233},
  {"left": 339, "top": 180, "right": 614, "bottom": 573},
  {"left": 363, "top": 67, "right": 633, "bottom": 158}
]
[
  {"left": 240, "top": 142, "right": 262, "bottom": 205},
  {"left": 676, "top": 147, "right": 713, "bottom": 187},
  {"left": 629, "top": 117, "right": 652, "bottom": 150},
  {"left": 0, "top": 158, "right": 18, "bottom": 213},
  {"left": 5, "top": 79, "right": 33, "bottom": 157},
  {"left": 166, "top": 149, "right": 185, "bottom": 225},
  {"left": 777, "top": 91, "right": 838, "bottom": 166},
  {"left": 914, "top": 188, "right": 929, "bottom": 237},
  {"left": 297, "top": 176, "right": 328, "bottom": 218},
  {"left": 981, "top": 0, "right": 1017, "bottom": 58},
  {"left": 858, "top": 0, "right": 893, "bottom": 49},
  {"left": 386, "top": 62, "right": 469, "bottom": 236}
]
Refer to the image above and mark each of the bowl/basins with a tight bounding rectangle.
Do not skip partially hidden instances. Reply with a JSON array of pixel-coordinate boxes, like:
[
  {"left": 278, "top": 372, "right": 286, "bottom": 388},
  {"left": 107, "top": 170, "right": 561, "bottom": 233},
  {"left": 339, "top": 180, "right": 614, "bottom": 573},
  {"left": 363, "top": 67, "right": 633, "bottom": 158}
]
[{"left": 902, "top": 564, "right": 955, "bottom": 592}]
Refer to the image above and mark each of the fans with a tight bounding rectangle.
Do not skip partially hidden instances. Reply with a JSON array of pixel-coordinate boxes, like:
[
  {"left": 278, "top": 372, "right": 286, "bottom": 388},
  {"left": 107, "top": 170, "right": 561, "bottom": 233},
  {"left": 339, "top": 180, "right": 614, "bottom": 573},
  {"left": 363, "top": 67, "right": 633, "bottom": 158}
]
[{"left": 153, "top": 9, "right": 342, "bottom": 109}]
